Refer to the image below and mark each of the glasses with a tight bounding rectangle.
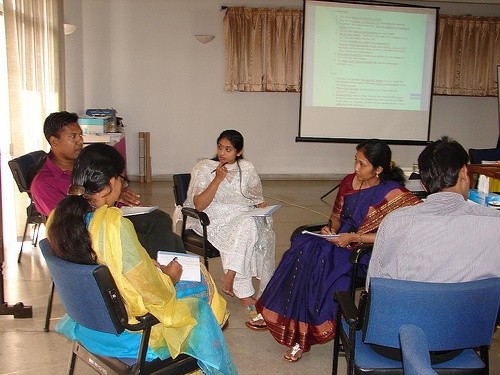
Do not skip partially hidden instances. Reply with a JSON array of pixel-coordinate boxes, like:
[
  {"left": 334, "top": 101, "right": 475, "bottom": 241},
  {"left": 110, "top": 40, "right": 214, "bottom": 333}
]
[{"left": 119, "top": 174, "right": 127, "bottom": 182}]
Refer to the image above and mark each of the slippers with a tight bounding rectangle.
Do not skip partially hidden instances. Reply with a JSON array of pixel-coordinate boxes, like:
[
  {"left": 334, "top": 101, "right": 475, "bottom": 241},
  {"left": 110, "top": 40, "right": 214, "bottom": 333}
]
[
  {"left": 247, "top": 304, "right": 257, "bottom": 317},
  {"left": 284, "top": 342, "right": 303, "bottom": 362},
  {"left": 245, "top": 313, "right": 268, "bottom": 330}
]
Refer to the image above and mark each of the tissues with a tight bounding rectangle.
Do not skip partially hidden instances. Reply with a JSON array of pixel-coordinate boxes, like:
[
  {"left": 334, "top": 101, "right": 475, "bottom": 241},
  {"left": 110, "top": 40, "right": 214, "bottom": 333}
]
[{"left": 468, "top": 174, "right": 499, "bottom": 207}]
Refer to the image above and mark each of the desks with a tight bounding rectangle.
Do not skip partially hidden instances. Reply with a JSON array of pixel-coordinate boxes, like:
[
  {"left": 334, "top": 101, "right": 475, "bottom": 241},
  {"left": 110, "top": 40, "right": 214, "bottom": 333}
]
[{"left": 82, "top": 132, "right": 126, "bottom": 162}]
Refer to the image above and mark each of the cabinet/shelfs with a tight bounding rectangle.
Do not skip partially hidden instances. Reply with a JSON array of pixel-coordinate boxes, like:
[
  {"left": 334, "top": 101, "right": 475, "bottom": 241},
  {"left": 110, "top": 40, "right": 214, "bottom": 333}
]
[{"left": 467, "top": 164, "right": 500, "bottom": 191}]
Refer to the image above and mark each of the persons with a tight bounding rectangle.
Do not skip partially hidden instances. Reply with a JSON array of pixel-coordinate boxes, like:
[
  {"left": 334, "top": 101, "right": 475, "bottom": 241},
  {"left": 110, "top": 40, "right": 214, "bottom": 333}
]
[
  {"left": 44, "top": 143, "right": 240, "bottom": 375},
  {"left": 182, "top": 129, "right": 275, "bottom": 310},
  {"left": 29, "top": 111, "right": 186, "bottom": 261},
  {"left": 243, "top": 139, "right": 422, "bottom": 362},
  {"left": 361, "top": 134, "right": 500, "bottom": 365}
]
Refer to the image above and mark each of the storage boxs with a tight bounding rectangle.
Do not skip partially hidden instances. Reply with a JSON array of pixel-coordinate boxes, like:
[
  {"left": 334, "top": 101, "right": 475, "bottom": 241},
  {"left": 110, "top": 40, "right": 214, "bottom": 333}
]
[{"left": 77, "top": 118, "right": 108, "bottom": 135}]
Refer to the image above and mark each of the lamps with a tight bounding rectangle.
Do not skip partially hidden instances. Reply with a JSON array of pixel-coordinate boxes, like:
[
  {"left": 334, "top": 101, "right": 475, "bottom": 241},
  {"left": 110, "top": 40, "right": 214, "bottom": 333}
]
[
  {"left": 64, "top": 24, "right": 78, "bottom": 34},
  {"left": 195, "top": 35, "right": 214, "bottom": 44}
]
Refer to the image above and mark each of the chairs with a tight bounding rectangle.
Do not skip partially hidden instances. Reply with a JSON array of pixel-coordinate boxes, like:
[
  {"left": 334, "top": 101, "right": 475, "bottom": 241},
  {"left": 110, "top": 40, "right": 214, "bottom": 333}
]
[
  {"left": 333, "top": 277, "right": 500, "bottom": 375},
  {"left": 9, "top": 151, "right": 47, "bottom": 263},
  {"left": 39, "top": 238, "right": 200, "bottom": 375},
  {"left": 172, "top": 173, "right": 220, "bottom": 272},
  {"left": 469, "top": 148, "right": 500, "bottom": 164},
  {"left": 289, "top": 220, "right": 374, "bottom": 303}
]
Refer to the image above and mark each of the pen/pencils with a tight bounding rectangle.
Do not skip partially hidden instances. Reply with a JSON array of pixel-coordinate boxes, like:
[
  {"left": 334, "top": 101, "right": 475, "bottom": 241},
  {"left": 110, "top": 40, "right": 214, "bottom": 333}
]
[
  {"left": 328, "top": 220, "right": 333, "bottom": 233},
  {"left": 210, "top": 162, "right": 229, "bottom": 174}
]
